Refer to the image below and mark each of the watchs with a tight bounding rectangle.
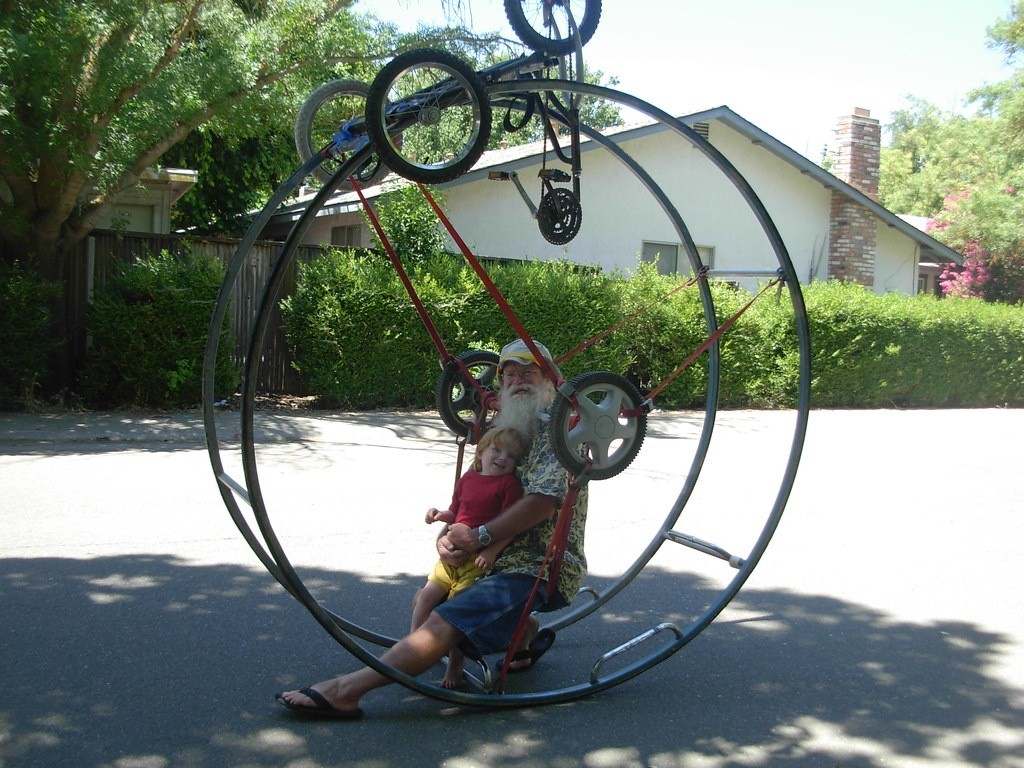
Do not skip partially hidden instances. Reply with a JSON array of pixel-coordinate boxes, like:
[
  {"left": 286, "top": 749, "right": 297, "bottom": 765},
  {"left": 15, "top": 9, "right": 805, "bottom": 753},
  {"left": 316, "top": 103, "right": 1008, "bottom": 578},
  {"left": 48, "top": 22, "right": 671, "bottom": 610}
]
[{"left": 479, "top": 525, "right": 491, "bottom": 546}]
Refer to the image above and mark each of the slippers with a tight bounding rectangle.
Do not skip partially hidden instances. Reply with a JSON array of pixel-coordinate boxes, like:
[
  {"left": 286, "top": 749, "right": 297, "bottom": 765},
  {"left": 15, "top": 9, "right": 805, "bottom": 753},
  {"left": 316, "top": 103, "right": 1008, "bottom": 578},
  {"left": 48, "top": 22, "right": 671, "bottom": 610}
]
[
  {"left": 276, "top": 685, "right": 364, "bottom": 720},
  {"left": 496, "top": 627, "right": 555, "bottom": 673}
]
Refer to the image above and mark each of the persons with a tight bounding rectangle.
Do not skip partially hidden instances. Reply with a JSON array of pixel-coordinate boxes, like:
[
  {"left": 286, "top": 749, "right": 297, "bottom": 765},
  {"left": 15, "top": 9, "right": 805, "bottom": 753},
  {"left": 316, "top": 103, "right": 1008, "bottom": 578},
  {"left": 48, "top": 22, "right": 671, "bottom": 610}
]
[
  {"left": 273, "top": 340, "right": 589, "bottom": 720},
  {"left": 411, "top": 427, "right": 522, "bottom": 689}
]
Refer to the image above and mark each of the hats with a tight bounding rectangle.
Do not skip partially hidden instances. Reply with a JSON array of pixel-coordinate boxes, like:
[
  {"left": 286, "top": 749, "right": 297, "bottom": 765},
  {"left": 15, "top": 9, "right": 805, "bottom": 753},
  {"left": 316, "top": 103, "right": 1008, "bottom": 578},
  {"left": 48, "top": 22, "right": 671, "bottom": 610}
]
[{"left": 493, "top": 338, "right": 553, "bottom": 396}]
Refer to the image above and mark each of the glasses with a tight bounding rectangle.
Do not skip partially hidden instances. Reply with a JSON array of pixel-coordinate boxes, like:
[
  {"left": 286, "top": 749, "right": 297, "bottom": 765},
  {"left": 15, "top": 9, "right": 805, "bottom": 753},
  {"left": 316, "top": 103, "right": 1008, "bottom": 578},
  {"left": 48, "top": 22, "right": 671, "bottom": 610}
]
[{"left": 500, "top": 369, "right": 542, "bottom": 385}]
